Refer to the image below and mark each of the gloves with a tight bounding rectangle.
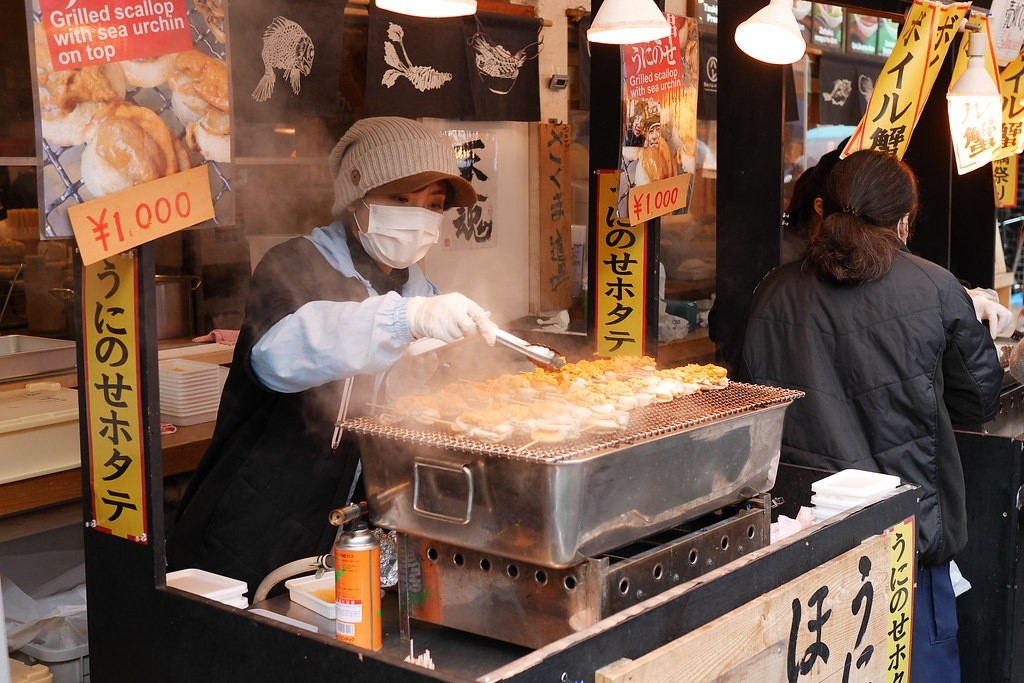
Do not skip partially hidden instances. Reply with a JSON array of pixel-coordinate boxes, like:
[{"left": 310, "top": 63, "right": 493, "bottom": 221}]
[
  {"left": 406, "top": 292, "right": 495, "bottom": 347},
  {"left": 972, "top": 296, "right": 1015, "bottom": 339}
]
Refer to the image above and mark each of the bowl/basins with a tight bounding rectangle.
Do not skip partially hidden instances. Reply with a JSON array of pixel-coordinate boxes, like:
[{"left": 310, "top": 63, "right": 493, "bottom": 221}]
[{"left": 793, "top": 0, "right": 900, "bottom": 42}]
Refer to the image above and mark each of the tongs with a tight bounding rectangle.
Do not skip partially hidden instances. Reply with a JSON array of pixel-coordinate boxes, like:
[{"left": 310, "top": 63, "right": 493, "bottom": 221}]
[{"left": 476, "top": 319, "right": 566, "bottom": 372}]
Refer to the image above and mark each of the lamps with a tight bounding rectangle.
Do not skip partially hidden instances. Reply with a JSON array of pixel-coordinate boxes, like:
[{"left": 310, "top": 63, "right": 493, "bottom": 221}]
[
  {"left": 942, "top": 20, "right": 1002, "bottom": 103},
  {"left": 734, "top": 0, "right": 806, "bottom": 65},
  {"left": 374, "top": 0, "right": 478, "bottom": 18},
  {"left": 586, "top": 0, "right": 671, "bottom": 44}
]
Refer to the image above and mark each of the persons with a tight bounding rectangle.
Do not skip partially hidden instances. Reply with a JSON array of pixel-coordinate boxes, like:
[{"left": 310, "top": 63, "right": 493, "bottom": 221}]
[
  {"left": 622, "top": 100, "right": 673, "bottom": 186},
  {"left": 161, "top": 116, "right": 499, "bottom": 606},
  {"left": 708, "top": 135, "right": 1013, "bottom": 683}
]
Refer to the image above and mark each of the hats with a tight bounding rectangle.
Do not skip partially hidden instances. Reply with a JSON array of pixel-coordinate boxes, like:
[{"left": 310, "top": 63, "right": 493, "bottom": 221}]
[{"left": 328, "top": 117, "right": 478, "bottom": 218}]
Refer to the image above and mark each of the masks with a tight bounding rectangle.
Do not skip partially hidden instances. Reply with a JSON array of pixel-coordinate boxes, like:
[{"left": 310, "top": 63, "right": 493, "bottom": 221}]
[{"left": 354, "top": 199, "right": 444, "bottom": 269}]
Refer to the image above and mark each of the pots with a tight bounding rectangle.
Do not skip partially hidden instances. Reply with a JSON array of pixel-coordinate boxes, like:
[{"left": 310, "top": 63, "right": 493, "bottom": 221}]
[{"left": 153, "top": 273, "right": 204, "bottom": 342}]
